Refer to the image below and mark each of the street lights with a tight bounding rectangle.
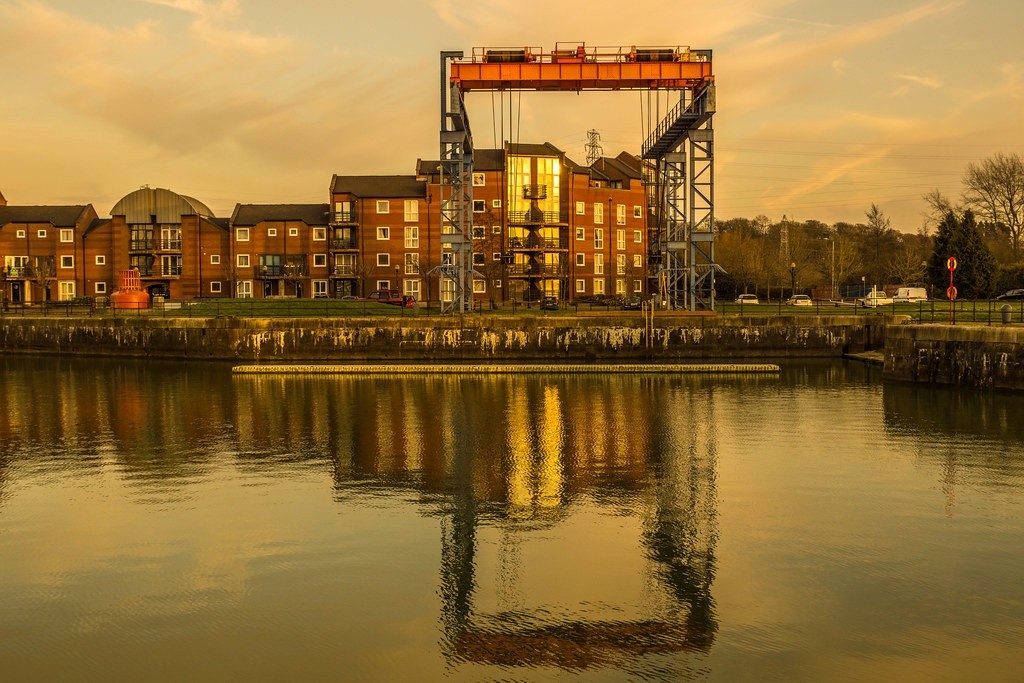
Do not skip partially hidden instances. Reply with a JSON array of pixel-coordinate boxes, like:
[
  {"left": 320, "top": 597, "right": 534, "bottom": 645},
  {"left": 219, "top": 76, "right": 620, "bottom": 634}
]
[
  {"left": 791, "top": 262, "right": 796, "bottom": 296},
  {"left": 824, "top": 237, "right": 834, "bottom": 298},
  {"left": 262, "top": 266, "right": 268, "bottom": 301},
  {"left": 395, "top": 265, "right": 399, "bottom": 289}
]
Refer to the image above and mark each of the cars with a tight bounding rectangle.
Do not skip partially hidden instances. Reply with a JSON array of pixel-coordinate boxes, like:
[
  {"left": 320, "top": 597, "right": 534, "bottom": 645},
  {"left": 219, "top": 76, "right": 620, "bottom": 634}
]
[
  {"left": 624, "top": 296, "right": 646, "bottom": 310},
  {"left": 735, "top": 294, "right": 759, "bottom": 304},
  {"left": 787, "top": 294, "right": 811, "bottom": 306},
  {"left": 540, "top": 299, "right": 558, "bottom": 310},
  {"left": 404, "top": 296, "right": 416, "bottom": 308},
  {"left": 997, "top": 289, "right": 1024, "bottom": 301},
  {"left": 367, "top": 289, "right": 405, "bottom": 305}
]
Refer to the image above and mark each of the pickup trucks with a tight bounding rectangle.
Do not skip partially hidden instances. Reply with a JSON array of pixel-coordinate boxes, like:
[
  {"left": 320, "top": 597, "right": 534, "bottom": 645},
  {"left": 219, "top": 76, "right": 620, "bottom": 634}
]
[{"left": 865, "top": 291, "right": 894, "bottom": 305}]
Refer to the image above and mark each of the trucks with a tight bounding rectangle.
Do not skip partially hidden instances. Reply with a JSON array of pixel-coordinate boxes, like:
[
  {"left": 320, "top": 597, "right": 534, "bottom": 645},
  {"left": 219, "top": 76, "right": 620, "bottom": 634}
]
[{"left": 892, "top": 288, "right": 928, "bottom": 303}]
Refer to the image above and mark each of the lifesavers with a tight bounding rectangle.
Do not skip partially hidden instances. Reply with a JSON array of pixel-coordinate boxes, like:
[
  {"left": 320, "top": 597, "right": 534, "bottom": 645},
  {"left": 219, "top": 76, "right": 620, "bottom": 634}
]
[
  {"left": 946, "top": 286, "right": 958, "bottom": 300},
  {"left": 946, "top": 257, "right": 957, "bottom": 272}
]
